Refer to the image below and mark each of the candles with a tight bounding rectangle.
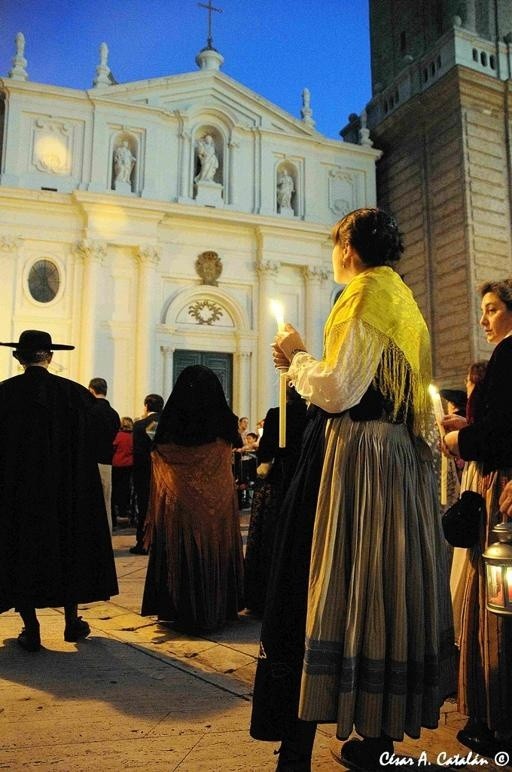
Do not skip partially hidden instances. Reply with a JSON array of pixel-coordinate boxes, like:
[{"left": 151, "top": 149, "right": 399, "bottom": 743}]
[
  {"left": 430, "top": 385, "right": 445, "bottom": 439},
  {"left": 441, "top": 443, "right": 448, "bottom": 505},
  {"left": 277, "top": 366, "right": 286, "bottom": 449},
  {"left": 269, "top": 307, "right": 284, "bottom": 333}
]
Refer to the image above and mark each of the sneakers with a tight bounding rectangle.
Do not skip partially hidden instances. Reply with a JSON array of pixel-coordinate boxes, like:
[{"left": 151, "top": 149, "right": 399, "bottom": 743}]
[{"left": 327, "top": 737, "right": 394, "bottom": 770}]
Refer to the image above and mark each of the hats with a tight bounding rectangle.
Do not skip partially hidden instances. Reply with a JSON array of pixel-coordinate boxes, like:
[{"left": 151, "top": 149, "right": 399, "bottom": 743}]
[
  {"left": 0, "top": 330, "right": 74, "bottom": 350},
  {"left": 440, "top": 390, "right": 467, "bottom": 411}
]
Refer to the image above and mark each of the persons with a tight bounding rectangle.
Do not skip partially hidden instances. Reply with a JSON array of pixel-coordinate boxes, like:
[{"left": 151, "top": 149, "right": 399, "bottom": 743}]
[
  {"left": 278, "top": 169, "right": 296, "bottom": 206},
  {"left": 0, "top": 207, "right": 512, "bottom": 772},
  {"left": 113, "top": 138, "right": 137, "bottom": 184},
  {"left": 194, "top": 134, "right": 219, "bottom": 183}
]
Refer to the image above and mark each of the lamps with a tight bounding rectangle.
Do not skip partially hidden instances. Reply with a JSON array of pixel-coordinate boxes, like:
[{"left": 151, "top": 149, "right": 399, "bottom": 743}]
[{"left": 481, "top": 515, "right": 512, "bottom": 616}]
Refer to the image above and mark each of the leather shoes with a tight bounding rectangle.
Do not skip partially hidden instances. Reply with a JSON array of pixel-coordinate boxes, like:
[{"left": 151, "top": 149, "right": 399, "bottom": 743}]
[
  {"left": 130, "top": 542, "right": 149, "bottom": 555},
  {"left": 17, "top": 630, "right": 40, "bottom": 650},
  {"left": 64, "top": 618, "right": 89, "bottom": 642}
]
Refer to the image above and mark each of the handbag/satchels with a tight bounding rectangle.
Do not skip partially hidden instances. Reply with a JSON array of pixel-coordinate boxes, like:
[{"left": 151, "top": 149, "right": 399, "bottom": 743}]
[{"left": 441, "top": 491, "right": 487, "bottom": 547}]
